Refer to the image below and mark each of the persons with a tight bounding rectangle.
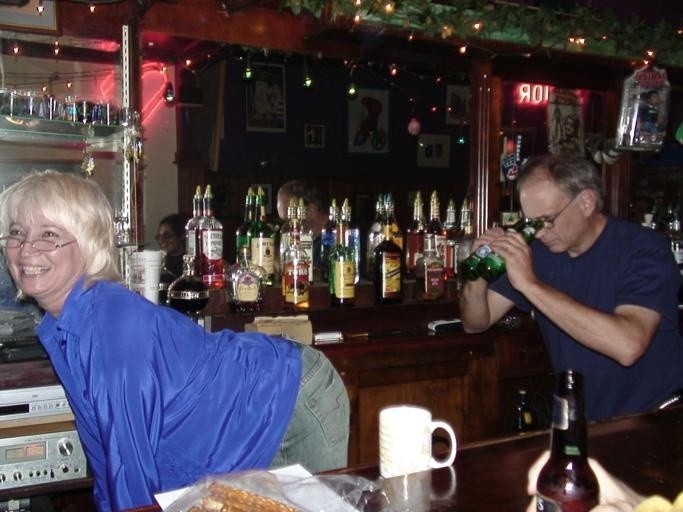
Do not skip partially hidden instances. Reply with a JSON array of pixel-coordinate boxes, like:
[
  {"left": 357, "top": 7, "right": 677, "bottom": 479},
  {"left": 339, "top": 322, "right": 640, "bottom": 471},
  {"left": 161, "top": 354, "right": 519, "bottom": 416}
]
[
  {"left": 0, "top": 170, "right": 351, "bottom": 512},
  {"left": 157, "top": 215, "right": 193, "bottom": 275},
  {"left": 460, "top": 155, "right": 683, "bottom": 423},
  {"left": 277, "top": 180, "right": 351, "bottom": 266},
  {"left": 641, "top": 95, "right": 661, "bottom": 136},
  {"left": 524, "top": 449, "right": 683, "bottom": 511}
]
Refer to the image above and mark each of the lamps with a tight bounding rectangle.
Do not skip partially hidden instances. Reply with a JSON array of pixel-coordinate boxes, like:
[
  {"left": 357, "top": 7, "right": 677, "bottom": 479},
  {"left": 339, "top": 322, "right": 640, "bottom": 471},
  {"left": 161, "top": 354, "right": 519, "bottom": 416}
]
[{"left": 13, "top": 2, "right": 682, "bottom": 144}]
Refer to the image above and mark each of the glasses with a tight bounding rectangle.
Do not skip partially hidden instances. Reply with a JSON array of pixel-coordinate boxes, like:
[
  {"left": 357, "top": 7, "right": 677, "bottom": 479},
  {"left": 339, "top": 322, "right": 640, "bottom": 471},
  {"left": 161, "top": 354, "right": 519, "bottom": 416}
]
[
  {"left": 154, "top": 232, "right": 179, "bottom": 241},
  {"left": 0, "top": 235, "right": 79, "bottom": 253},
  {"left": 518, "top": 189, "right": 585, "bottom": 232}
]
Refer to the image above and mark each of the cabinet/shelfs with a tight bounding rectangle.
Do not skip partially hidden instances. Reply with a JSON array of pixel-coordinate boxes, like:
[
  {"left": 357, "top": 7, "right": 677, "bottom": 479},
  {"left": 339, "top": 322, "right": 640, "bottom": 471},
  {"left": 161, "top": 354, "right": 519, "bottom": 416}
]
[
  {"left": 1, "top": 26, "right": 145, "bottom": 307},
  {"left": 196, "top": 282, "right": 551, "bottom": 470}
]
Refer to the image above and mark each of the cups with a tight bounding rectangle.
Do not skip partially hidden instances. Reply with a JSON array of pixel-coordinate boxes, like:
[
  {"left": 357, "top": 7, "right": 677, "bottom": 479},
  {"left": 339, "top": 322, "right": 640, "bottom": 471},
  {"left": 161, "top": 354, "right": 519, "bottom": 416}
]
[
  {"left": 378, "top": 468, "right": 458, "bottom": 510},
  {"left": 379, "top": 406, "right": 457, "bottom": 478}
]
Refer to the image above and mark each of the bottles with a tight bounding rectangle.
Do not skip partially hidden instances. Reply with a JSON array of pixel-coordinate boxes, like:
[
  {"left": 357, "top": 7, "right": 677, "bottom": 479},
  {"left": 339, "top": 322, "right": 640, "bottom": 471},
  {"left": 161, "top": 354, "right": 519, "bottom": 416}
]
[
  {"left": 253, "top": 188, "right": 278, "bottom": 284},
  {"left": 515, "top": 390, "right": 541, "bottom": 435},
  {"left": 406, "top": 190, "right": 423, "bottom": 267},
  {"left": 226, "top": 238, "right": 268, "bottom": 314},
  {"left": 368, "top": 196, "right": 383, "bottom": 271},
  {"left": 446, "top": 200, "right": 460, "bottom": 243},
  {"left": 332, "top": 194, "right": 358, "bottom": 304},
  {"left": 236, "top": 188, "right": 255, "bottom": 269},
  {"left": 534, "top": 373, "right": 600, "bottom": 508},
  {"left": 658, "top": 205, "right": 683, "bottom": 267},
  {"left": 281, "top": 207, "right": 310, "bottom": 312},
  {"left": 633, "top": 212, "right": 657, "bottom": 228},
  {"left": 462, "top": 208, "right": 476, "bottom": 271},
  {"left": 281, "top": 201, "right": 296, "bottom": 250},
  {"left": 168, "top": 252, "right": 211, "bottom": 313},
  {"left": 377, "top": 194, "right": 404, "bottom": 307},
  {"left": 422, "top": 193, "right": 447, "bottom": 303},
  {"left": 478, "top": 216, "right": 540, "bottom": 284},
  {"left": 461, "top": 216, "right": 538, "bottom": 282},
  {"left": 187, "top": 187, "right": 198, "bottom": 277},
  {"left": 200, "top": 185, "right": 226, "bottom": 291},
  {"left": 322, "top": 201, "right": 334, "bottom": 297}
]
